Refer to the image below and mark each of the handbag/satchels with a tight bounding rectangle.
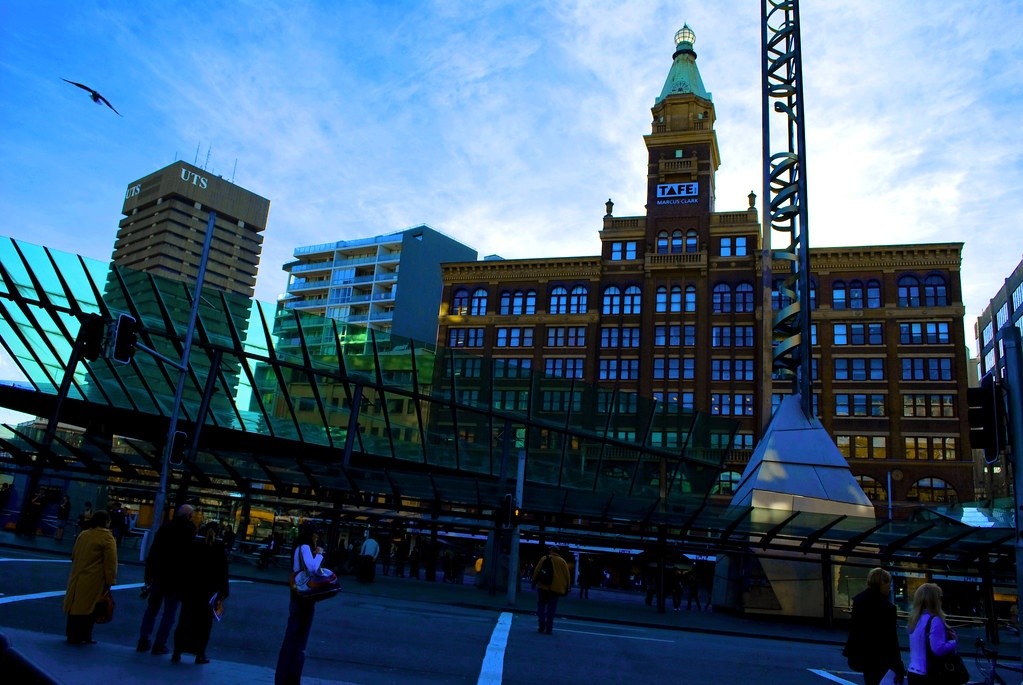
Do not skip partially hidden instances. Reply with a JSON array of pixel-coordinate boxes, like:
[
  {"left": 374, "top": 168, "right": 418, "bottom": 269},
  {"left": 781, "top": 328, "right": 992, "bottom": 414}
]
[
  {"left": 924, "top": 615, "right": 969, "bottom": 685},
  {"left": 94, "top": 585, "right": 115, "bottom": 624},
  {"left": 301, "top": 567, "right": 341, "bottom": 603}
]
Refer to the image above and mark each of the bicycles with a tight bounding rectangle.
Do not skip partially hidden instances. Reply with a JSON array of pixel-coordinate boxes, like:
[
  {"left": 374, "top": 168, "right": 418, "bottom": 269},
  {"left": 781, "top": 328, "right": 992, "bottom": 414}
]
[{"left": 967, "top": 636, "right": 1023, "bottom": 684}]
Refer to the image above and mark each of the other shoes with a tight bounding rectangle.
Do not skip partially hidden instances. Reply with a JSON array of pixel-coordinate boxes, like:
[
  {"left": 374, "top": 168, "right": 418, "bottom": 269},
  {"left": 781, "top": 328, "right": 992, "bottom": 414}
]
[
  {"left": 171, "top": 655, "right": 181, "bottom": 663},
  {"left": 151, "top": 644, "right": 169, "bottom": 655},
  {"left": 136, "top": 639, "right": 152, "bottom": 653},
  {"left": 195, "top": 656, "right": 209, "bottom": 663}
]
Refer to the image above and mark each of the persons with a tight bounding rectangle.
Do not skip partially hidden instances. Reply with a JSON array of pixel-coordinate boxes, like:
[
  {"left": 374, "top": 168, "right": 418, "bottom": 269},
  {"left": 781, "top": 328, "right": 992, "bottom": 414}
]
[
  {"left": 63, "top": 510, "right": 118, "bottom": 644},
  {"left": 842, "top": 566, "right": 905, "bottom": 685},
  {"left": 0, "top": 482, "right": 11, "bottom": 515},
  {"left": 262, "top": 530, "right": 282, "bottom": 569},
  {"left": 670, "top": 571, "right": 702, "bottom": 612},
  {"left": 6, "top": 484, "right": 17, "bottom": 510},
  {"left": 54, "top": 495, "right": 72, "bottom": 540},
  {"left": 339, "top": 529, "right": 422, "bottom": 584},
  {"left": 645, "top": 573, "right": 656, "bottom": 607},
  {"left": 532, "top": 545, "right": 571, "bottom": 635},
  {"left": 24, "top": 485, "right": 49, "bottom": 541},
  {"left": 77, "top": 500, "right": 130, "bottom": 551},
  {"left": 519, "top": 561, "right": 537, "bottom": 588},
  {"left": 907, "top": 583, "right": 960, "bottom": 685},
  {"left": 274, "top": 522, "right": 325, "bottom": 684},
  {"left": 136, "top": 503, "right": 236, "bottom": 664}
]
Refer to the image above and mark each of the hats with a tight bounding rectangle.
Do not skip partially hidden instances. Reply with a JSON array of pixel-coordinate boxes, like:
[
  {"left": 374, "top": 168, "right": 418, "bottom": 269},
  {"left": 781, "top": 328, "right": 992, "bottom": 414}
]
[{"left": 548, "top": 545, "right": 560, "bottom": 551}]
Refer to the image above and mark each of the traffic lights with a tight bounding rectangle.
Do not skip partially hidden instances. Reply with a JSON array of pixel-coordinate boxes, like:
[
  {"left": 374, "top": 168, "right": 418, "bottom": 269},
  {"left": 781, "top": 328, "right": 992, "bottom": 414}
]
[
  {"left": 172, "top": 433, "right": 191, "bottom": 466},
  {"left": 150, "top": 441, "right": 163, "bottom": 468},
  {"left": 77, "top": 314, "right": 90, "bottom": 358},
  {"left": 119, "top": 318, "right": 138, "bottom": 361},
  {"left": 501, "top": 499, "right": 509, "bottom": 523},
  {"left": 966, "top": 386, "right": 991, "bottom": 450},
  {"left": 514, "top": 507, "right": 521, "bottom": 520}
]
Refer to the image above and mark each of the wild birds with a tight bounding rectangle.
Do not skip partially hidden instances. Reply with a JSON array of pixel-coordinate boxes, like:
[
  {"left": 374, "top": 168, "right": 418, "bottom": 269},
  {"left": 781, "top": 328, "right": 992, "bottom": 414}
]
[{"left": 58, "top": 76, "right": 122, "bottom": 115}]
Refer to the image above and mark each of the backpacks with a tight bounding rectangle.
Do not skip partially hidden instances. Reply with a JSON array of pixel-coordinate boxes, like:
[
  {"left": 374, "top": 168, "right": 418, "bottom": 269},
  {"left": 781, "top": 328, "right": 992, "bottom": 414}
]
[{"left": 538, "top": 555, "right": 554, "bottom": 588}]
